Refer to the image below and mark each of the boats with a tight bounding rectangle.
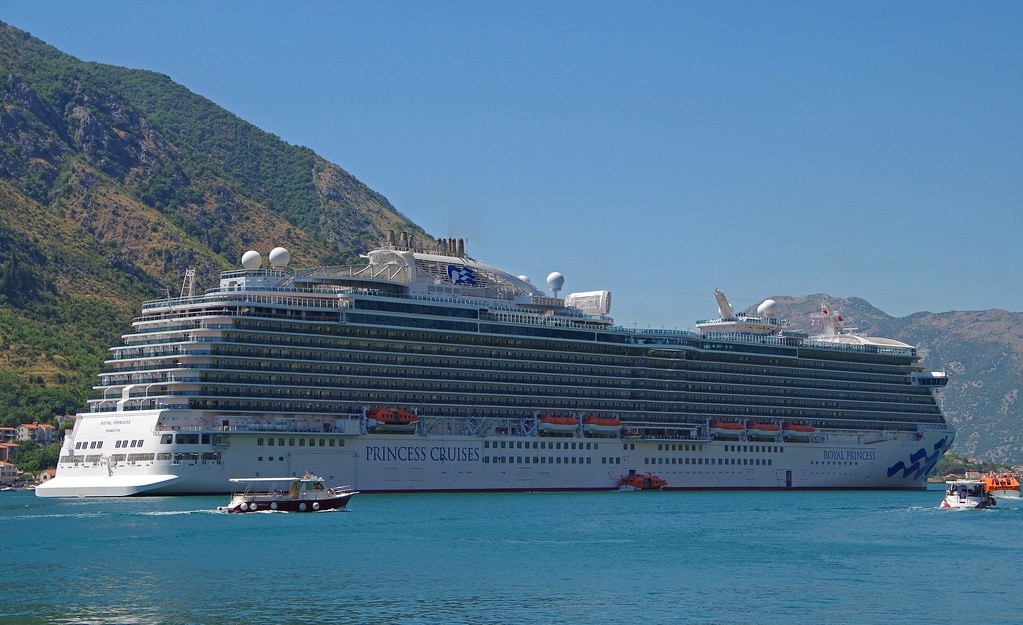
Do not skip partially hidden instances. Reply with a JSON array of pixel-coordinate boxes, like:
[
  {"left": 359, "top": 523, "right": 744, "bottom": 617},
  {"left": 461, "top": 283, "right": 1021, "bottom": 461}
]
[
  {"left": 979, "top": 469, "right": 1020, "bottom": 497},
  {"left": 2, "top": 474, "right": 41, "bottom": 493},
  {"left": 618, "top": 472, "right": 668, "bottom": 492},
  {"left": 940, "top": 477, "right": 997, "bottom": 509},
  {"left": 223, "top": 470, "right": 363, "bottom": 514}
]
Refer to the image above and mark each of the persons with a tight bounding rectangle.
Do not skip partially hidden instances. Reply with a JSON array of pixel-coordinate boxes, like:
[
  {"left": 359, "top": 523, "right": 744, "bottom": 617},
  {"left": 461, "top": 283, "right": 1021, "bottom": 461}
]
[{"left": 990, "top": 470, "right": 998, "bottom": 478}]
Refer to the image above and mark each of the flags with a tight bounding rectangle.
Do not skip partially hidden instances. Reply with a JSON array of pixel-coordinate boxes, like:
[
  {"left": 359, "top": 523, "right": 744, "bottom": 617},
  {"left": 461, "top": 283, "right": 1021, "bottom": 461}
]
[{"left": 447, "top": 264, "right": 477, "bottom": 285}]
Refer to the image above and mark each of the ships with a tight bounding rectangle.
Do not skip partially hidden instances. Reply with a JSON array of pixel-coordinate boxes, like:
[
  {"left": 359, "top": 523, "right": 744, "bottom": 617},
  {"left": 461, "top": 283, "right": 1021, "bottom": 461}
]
[{"left": 36, "top": 231, "right": 957, "bottom": 499}]
